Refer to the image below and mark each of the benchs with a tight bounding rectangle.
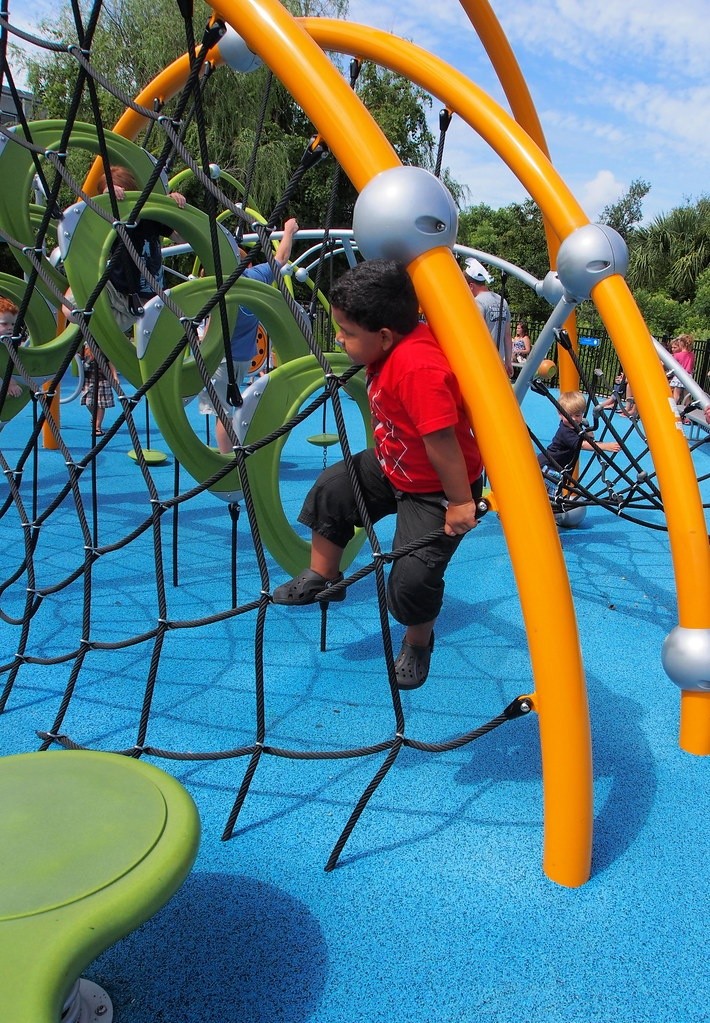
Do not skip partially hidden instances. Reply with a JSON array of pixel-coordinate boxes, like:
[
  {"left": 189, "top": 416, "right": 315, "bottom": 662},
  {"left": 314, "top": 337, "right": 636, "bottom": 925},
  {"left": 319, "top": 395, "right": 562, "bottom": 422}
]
[{"left": 676, "top": 392, "right": 710, "bottom": 439}]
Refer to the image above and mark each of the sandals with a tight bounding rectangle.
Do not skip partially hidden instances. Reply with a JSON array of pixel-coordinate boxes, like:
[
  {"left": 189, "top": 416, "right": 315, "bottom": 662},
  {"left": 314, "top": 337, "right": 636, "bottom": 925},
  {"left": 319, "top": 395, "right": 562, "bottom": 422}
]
[
  {"left": 394, "top": 629, "right": 435, "bottom": 689},
  {"left": 96, "top": 430, "right": 105, "bottom": 436},
  {"left": 273, "top": 567, "right": 346, "bottom": 605}
]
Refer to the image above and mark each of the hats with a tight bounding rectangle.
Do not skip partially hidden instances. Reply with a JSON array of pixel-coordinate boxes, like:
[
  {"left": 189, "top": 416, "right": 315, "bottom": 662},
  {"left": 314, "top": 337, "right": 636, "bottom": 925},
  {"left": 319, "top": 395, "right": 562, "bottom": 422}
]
[{"left": 465, "top": 257, "right": 494, "bottom": 283}]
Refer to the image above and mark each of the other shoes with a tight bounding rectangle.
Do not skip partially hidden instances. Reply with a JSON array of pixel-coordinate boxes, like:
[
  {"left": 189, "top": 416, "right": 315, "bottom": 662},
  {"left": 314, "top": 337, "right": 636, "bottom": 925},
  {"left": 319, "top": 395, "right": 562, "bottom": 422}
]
[
  {"left": 620, "top": 412, "right": 635, "bottom": 417},
  {"left": 616, "top": 408, "right": 626, "bottom": 413},
  {"left": 246, "top": 381, "right": 253, "bottom": 385},
  {"left": 604, "top": 405, "right": 614, "bottom": 410}
]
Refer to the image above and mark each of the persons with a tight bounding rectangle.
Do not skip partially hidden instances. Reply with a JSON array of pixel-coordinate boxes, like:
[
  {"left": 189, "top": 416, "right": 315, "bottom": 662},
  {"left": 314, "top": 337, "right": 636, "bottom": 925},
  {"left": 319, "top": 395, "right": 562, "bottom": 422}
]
[
  {"left": 62, "top": 168, "right": 186, "bottom": 331},
  {"left": 537, "top": 391, "right": 622, "bottom": 481},
  {"left": 511, "top": 322, "right": 530, "bottom": 360},
  {"left": 599, "top": 335, "right": 696, "bottom": 424},
  {"left": 79, "top": 341, "right": 120, "bottom": 436},
  {"left": 0, "top": 298, "right": 23, "bottom": 398},
  {"left": 197, "top": 219, "right": 298, "bottom": 452},
  {"left": 272, "top": 258, "right": 482, "bottom": 689},
  {"left": 463, "top": 266, "right": 514, "bottom": 378}
]
